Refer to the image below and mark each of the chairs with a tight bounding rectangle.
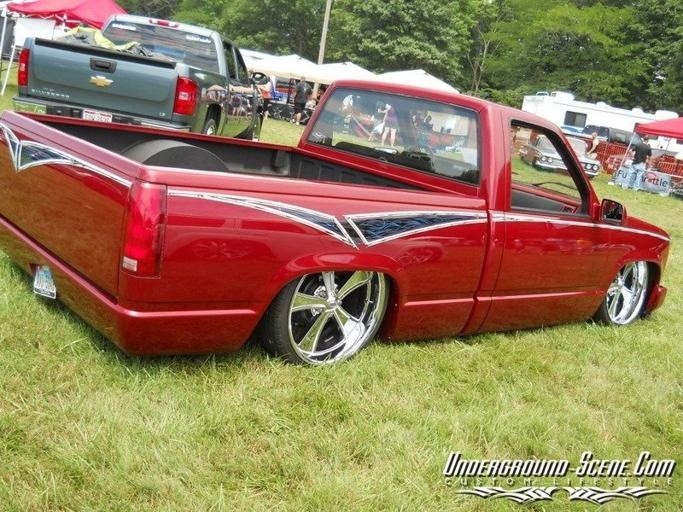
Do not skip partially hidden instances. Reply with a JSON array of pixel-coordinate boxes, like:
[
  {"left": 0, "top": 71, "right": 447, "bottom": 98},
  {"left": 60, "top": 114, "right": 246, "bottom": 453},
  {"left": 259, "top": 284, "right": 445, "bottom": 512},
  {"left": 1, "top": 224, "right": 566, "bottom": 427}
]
[{"left": 335, "top": 141, "right": 478, "bottom": 184}]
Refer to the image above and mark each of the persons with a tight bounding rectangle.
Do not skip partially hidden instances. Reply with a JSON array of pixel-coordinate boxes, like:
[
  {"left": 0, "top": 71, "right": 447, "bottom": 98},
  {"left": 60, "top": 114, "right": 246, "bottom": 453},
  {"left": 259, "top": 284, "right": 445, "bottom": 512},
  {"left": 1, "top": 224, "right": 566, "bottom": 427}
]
[
  {"left": 339, "top": 95, "right": 398, "bottom": 148},
  {"left": 204, "top": 90, "right": 252, "bottom": 118},
  {"left": 287, "top": 75, "right": 312, "bottom": 127},
  {"left": 248, "top": 67, "right": 271, "bottom": 143},
  {"left": 621, "top": 134, "right": 652, "bottom": 189},
  {"left": 586, "top": 132, "right": 599, "bottom": 160}
]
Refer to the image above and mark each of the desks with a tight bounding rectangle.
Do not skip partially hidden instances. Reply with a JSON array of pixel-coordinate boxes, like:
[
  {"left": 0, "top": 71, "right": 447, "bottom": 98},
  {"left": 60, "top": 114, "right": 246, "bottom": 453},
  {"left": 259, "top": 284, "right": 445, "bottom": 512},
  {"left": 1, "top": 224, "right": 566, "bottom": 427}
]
[{"left": 623, "top": 165, "right": 682, "bottom": 197}]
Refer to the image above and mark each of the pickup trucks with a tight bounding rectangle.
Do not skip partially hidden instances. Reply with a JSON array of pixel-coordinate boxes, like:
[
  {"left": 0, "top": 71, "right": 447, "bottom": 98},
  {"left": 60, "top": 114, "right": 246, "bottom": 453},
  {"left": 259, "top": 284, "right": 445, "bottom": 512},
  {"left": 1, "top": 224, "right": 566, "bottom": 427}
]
[
  {"left": 518, "top": 130, "right": 601, "bottom": 180},
  {"left": 12, "top": 12, "right": 271, "bottom": 144},
  {"left": 1, "top": 77, "right": 672, "bottom": 368}
]
